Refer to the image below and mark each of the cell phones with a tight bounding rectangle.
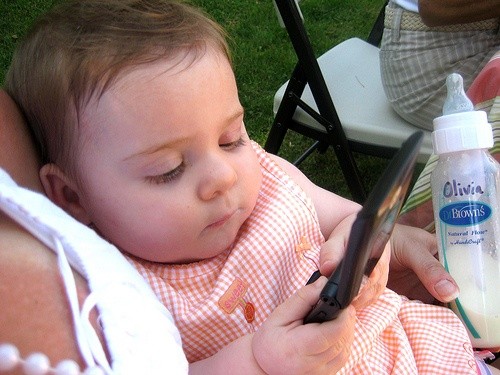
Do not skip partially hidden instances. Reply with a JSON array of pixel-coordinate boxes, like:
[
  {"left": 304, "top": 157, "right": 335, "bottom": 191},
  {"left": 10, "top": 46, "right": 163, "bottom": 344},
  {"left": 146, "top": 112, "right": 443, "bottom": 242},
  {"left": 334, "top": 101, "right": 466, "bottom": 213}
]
[{"left": 303, "top": 130, "right": 423, "bottom": 324}]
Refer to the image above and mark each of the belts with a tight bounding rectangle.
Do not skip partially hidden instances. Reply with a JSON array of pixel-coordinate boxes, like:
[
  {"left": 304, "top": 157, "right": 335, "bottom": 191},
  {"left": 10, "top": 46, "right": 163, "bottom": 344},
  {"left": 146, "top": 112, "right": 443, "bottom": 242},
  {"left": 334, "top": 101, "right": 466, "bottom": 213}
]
[{"left": 384, "top": 5, "right": 499, "bottom": 32}]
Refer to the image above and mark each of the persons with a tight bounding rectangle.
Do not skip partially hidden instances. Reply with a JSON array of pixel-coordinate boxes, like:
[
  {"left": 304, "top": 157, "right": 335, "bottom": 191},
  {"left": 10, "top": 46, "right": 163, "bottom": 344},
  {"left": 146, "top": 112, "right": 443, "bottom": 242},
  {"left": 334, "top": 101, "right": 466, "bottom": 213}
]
[
  {"left": 0, "top": 89, "right": 462, "bottom": 375},
  {"left": 4, "top": 1, "right": 499, "bottom": 375},
  {"left": 379, "top": 0, "right": 500, "bottom": 131}
]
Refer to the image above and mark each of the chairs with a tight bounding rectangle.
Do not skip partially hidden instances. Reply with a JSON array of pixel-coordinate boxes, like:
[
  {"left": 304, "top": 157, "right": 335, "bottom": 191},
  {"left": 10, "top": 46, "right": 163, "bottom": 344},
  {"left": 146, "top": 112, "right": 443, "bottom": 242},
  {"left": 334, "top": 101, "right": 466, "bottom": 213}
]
[{"left": 259, "top": 0, "right": 435, "bottom": 201}]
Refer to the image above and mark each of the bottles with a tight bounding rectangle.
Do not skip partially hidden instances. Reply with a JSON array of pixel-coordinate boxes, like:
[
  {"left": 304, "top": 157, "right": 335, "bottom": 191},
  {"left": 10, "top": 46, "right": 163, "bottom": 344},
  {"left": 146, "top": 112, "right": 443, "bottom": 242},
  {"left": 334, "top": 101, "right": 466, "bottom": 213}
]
[{"left": 431, "top": 71, "right": 499, "bottom": 350}]
